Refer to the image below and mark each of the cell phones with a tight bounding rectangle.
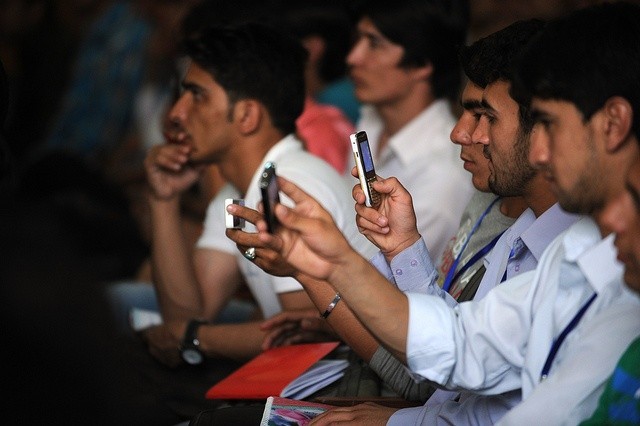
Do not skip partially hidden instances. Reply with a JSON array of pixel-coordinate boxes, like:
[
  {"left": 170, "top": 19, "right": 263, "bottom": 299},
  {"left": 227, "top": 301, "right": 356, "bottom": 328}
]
[
  {"left": 350, "top": 130, "right": 382, "bottom": 208},
  {"left": 260, "top": 161, "right": 280, "bottom": 234},
  {"left": 225, "top": 198, "right": 245, "bottom": 229}
]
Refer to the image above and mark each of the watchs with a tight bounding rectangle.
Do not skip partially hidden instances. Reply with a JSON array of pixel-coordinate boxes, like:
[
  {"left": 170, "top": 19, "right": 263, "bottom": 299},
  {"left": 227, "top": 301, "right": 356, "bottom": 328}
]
[{"left": 177, "top": 319, "right": 204, "bottom": 375}]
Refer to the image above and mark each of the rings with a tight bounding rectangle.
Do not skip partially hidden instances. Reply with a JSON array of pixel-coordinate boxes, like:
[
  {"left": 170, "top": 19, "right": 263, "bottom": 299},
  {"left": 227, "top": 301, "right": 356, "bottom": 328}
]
[{"left": 245, "top": 246, "right": 255, "bottom": 262}]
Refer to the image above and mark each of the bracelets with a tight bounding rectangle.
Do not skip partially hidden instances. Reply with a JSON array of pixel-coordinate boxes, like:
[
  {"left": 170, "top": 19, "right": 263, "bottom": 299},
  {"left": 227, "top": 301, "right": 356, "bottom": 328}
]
[{"left": 319, "top": 291, "right": 340, "bottom": 319}]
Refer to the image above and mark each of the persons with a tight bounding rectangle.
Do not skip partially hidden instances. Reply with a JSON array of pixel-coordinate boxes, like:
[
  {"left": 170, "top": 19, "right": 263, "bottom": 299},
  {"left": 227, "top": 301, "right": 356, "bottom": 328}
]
[
  {"left": 343, "top": 1, "right": 478, "bottom": 300},
  {"left": 302, "top": 15, "right": 585, "bottom": 421},
  {"left": 253, "top": 11, "right": 638, "bottom": 425},
  {"left": 224, "top": 21, "right": 529, "bottom": 420},
  {"left": 91, "top": 9, "right": 381, "bottom": 422},
  {"left": 293, "top": 12, "right": 368, "bottom": 126},
  {"left": 574, "top": 150, "right": 639, "bottom": 425}
]
